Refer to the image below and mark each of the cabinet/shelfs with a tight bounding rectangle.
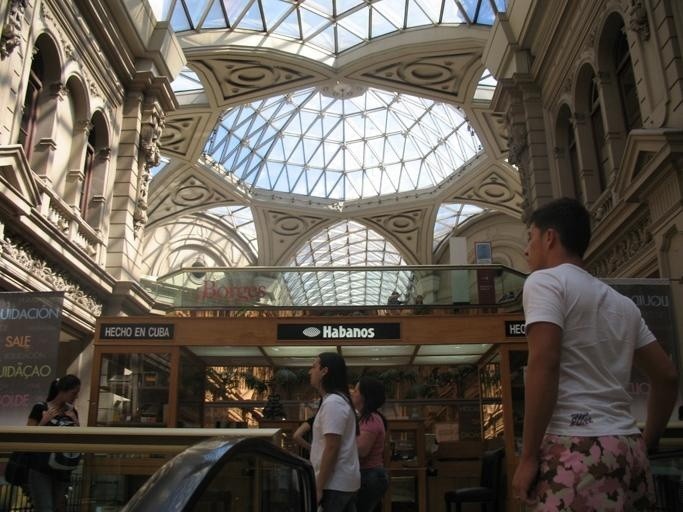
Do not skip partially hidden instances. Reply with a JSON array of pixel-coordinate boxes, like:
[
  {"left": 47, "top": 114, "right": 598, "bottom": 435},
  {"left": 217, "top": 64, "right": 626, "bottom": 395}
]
[
  {"left": 260, "top": 420, "right": 302, "bottom": 457},
  {"left": 474, "top": 338, "right": 537, "bottom": 512},
  {"left": 384, "top": 419, "right": 425, "bottom": 465},
  {"left": 80, "top": 345, "right": 205, "bottom": 512}
]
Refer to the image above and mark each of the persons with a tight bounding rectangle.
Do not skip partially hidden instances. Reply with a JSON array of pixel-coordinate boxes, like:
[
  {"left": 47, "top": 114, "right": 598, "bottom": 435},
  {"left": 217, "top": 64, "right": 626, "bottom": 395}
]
[
  {"left": 308, "top": 350, "right": 362, "bottom": 512},
  {"left": 292, "top": 414, "right": 315, "bottom": 450},
  {"left": 4, "top": 373, "right": 83, "bottom": 512},
  {"left": 351, "top": 375, "right": 392, "bottom": 512},
  {"left": 510, "top": 195, "right": 679, "bottom": 511}
]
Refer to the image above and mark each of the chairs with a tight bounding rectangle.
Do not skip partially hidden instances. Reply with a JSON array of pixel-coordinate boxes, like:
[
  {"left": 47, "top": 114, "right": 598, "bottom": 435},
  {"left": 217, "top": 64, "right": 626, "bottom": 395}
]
[{"left": 444, "top": 446, "right": 505, "bottom": 512}]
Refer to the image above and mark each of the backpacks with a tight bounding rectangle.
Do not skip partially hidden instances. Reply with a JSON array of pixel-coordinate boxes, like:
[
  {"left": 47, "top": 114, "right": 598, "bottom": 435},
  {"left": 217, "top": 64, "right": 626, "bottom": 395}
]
[{"left": 40, "top": 401, "right": 82, "bottom": 471}]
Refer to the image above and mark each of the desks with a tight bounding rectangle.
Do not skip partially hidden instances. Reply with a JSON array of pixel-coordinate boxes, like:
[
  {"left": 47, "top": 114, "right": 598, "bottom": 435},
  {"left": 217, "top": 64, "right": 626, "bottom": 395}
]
[{"left": 383, "top": 466, "right": 437, "bottom": 512}]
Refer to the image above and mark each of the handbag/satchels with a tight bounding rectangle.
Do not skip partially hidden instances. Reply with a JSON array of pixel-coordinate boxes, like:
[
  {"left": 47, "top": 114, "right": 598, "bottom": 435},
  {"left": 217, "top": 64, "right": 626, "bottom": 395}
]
[{"left": 4, "top": 453, "right": 27, "bottom": 485}]
